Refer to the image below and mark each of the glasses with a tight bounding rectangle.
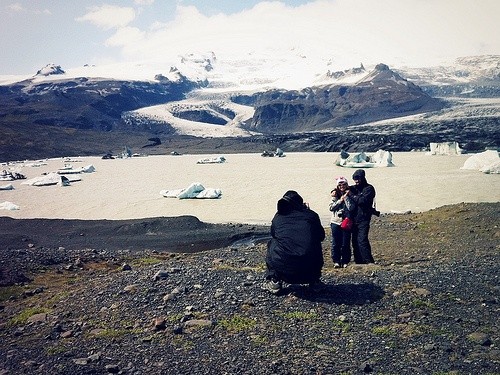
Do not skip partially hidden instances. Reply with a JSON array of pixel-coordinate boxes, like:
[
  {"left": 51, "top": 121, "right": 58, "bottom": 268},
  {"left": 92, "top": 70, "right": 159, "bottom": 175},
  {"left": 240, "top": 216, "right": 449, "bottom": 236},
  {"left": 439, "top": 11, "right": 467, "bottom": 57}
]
[{"left": 338, "top": 183, "right": 347, "bottom": 186}]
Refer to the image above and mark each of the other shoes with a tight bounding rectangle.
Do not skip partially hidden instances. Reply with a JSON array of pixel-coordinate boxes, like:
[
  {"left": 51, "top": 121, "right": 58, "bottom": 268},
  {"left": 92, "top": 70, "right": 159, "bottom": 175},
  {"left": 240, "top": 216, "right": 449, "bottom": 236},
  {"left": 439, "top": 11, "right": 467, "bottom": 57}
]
[
  {"left": 333, "top": 262, "right": 340, "bottom": 268},
  {"left": 342, "top": 264, "right": 347, "bottom": 268}
]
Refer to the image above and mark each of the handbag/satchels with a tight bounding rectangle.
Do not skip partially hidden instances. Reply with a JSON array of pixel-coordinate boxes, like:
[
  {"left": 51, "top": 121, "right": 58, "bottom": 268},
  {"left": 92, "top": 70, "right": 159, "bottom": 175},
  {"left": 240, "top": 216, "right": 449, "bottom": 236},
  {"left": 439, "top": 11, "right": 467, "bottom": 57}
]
[{"left": 341, "top": 217, "right": 353, "bottom": 230}]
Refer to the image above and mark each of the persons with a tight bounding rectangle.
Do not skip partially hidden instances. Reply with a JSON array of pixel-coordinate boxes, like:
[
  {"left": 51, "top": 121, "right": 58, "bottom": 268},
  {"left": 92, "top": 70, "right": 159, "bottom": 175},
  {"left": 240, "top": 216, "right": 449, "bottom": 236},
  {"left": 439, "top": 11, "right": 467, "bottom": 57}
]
[
  {"left": 329, "top": 177, "right": 352, "bottom": 268},
  {"left": 262, "top": 190, "right": 325, "bottom": 294},
  {"left": 331, "top": 169, "right": 375, "bottom": 264}
]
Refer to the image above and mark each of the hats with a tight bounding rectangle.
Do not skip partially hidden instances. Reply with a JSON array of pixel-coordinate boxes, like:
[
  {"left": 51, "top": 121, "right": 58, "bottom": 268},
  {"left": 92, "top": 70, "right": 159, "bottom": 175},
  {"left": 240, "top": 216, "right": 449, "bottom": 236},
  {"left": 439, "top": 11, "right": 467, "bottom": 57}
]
[
  {"left": 337, "top": 177, "right": 348, "bottom": 186},
  {"left": 353, "top": 169, "right": 365, "bottom": 180}
]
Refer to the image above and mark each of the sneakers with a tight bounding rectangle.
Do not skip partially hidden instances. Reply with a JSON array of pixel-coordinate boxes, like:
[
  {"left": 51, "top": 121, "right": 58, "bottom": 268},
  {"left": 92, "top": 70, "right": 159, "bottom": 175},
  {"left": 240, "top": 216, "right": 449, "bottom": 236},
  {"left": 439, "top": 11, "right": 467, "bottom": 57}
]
[
  {"left": 310, "top": 281, "right": 325, "bottom": 291},
  {"left": 260, "top": 280, "right": 282, "bottom": 292}
]
